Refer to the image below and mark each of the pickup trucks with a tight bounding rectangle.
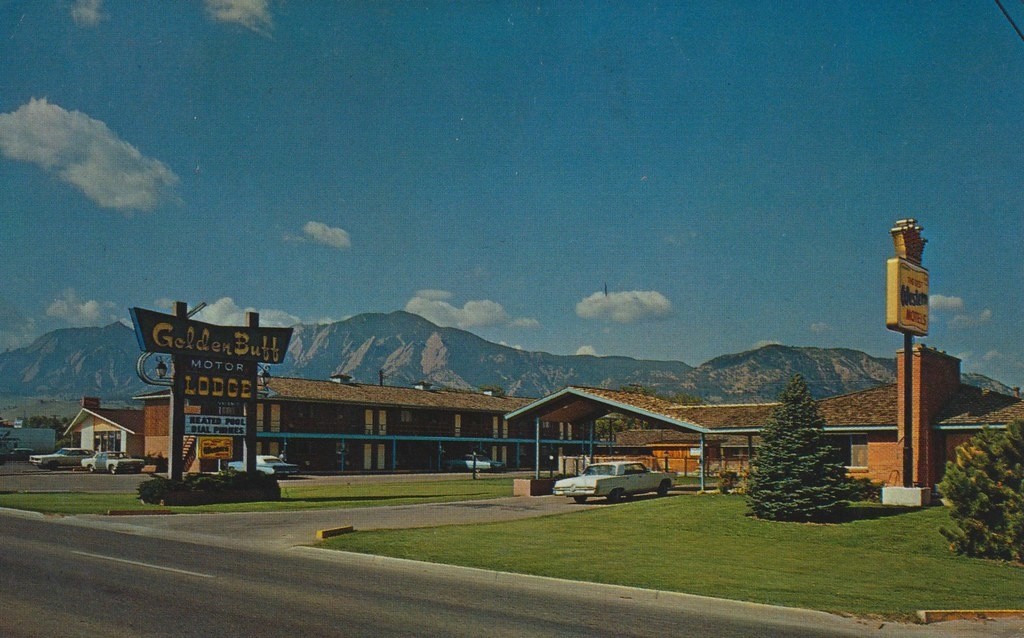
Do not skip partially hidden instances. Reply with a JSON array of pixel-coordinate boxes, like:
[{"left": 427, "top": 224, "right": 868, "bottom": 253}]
[{"left": 554, "top": 460, "right": 679, "bottom": 502}]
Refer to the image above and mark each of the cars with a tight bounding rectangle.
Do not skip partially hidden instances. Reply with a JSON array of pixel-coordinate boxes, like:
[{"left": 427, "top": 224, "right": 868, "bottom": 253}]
[
  {"left": 29, "top": 447, "right": 98, "bottom": 472},
  {"left": 446, "top": 453, "right": 504, "bottom": 471},
  {"left": 221, "top": 454, "right": 296, "bottom": 480},
  {"left": 83, "top": 451, "right": 145, "bottom": 471}
]
